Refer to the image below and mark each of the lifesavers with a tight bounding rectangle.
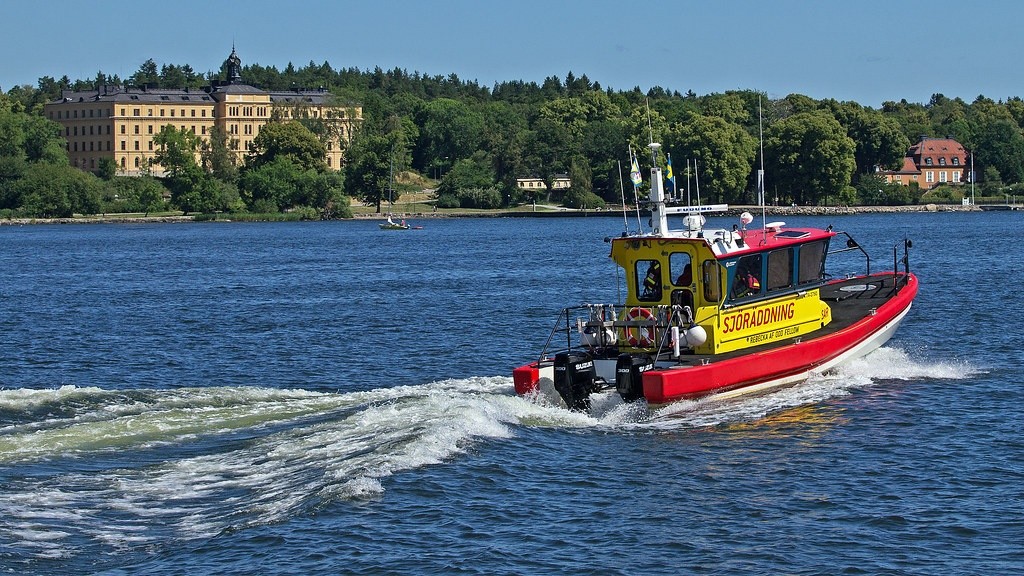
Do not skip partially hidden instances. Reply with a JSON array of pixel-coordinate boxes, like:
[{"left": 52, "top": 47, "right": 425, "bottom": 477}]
[{"left": 623, "top": 307, "right": 657, "bottom": 347}]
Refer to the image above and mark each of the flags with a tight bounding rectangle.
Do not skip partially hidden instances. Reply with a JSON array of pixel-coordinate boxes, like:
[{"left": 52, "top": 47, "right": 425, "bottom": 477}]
[
  {"left": 629, "top": 157, "right": 642, "bottom": 187},
  {"left": 664, "top": 157, "right": 673, "bottom": 193}
]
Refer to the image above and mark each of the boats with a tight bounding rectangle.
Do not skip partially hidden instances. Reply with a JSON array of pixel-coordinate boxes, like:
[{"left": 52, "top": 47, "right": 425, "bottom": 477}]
[
  {"left": 378, "top": 213, "right": 416, "bottom": 230},
  {"left": 513, "top": 95, "right": 921, "bottom": 416}
]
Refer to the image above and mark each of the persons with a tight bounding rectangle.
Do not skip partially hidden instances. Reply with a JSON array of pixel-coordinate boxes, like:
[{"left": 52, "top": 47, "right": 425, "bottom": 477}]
[
  {"left": 676, "top": 264, "right": 692, "bottom": 287},
  {"left": 401, "top": 219, "right": 405, "bottom": 226},
  {"left": 387, "top": 215, "right": 394, "bottom": 224},
  {"left": 729, "top": 261, "right": 760, "bottom": 298}
]
